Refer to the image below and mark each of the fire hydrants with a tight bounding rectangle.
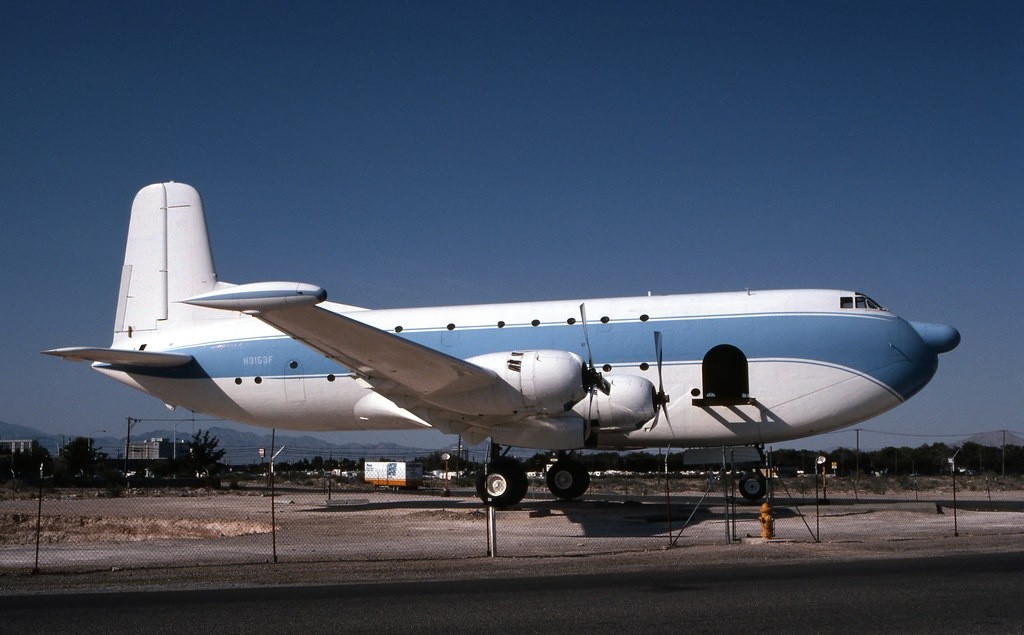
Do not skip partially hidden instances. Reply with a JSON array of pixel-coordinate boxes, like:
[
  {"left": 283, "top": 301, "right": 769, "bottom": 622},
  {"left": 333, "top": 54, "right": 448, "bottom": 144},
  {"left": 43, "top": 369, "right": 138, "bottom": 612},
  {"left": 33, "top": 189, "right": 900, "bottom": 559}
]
[{"left": 758, "top": 502, "right": 777, "bottom": 541}]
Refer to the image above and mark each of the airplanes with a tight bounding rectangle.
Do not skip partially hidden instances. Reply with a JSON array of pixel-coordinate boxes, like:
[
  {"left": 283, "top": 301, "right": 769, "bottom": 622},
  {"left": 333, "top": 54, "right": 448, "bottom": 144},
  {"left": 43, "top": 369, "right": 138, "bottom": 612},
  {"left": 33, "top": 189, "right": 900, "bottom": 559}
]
[{"left": 36, "top": 178, "right": 963, "bottom": 510}]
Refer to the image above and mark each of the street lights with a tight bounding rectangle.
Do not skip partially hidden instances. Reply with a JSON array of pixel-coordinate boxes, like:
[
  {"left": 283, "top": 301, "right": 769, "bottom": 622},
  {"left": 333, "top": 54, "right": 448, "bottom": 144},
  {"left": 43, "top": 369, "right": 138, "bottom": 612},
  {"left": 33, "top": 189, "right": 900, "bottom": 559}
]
[{"left": 88, "top": 430, "right": 106, "bottom": 451}]
